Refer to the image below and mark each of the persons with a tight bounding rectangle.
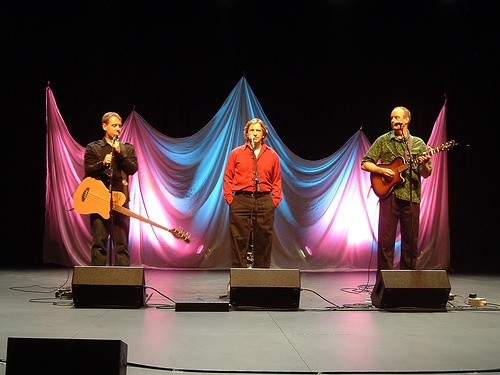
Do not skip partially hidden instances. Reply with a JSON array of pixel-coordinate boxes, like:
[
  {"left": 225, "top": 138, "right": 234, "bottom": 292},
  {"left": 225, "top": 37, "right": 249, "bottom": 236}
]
[
  {"left": 83, "top": 111, "right": 139, "bottom": 266},
  {"left": 360, "top": 105, "right": 433, "bottom": 281},
  {"left": 223, "top": 118, "right": 283, "bottom": 268}
]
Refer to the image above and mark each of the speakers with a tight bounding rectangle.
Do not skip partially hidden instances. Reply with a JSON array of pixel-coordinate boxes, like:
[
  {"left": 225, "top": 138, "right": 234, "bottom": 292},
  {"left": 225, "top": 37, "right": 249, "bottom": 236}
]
[
  {"left": 229, "top": 268, "right": 300, "bottom": 309},
  {"left": 5, "top": 337, "right": 128, "bottom": 375},
  {"left": 72, "top": 266, "right": 146, "bottom": 310},
  {"left": 370, "top": 269, "right": 452, "bottom": 309}
]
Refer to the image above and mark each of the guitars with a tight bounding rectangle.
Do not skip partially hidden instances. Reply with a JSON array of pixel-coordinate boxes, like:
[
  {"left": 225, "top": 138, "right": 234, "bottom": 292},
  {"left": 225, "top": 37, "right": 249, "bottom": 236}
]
[
  {"left": 72, "top": 176, "right": 193, "bottom": 244},
  {"left": 370, "top": 137, "right": 459, "bottom": 198}
]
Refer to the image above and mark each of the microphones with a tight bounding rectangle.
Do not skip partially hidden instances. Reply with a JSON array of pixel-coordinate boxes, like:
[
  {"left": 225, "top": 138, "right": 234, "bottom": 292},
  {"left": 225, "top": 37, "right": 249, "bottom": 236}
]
[
  {"left": 394, "top": 121, "right": 403, "bottom": 126},
  {"left": 113, "top": 132, "right": 119, "bottom": 143},
  {"left": 250, "top": 135, "right": 255, "bottom": 148}
]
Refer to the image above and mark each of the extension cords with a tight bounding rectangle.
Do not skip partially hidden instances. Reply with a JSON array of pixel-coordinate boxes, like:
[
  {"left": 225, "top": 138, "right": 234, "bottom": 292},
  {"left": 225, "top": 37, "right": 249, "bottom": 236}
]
[{"left": 468, "top": 297, "right": 486, "bottom": 307}]
